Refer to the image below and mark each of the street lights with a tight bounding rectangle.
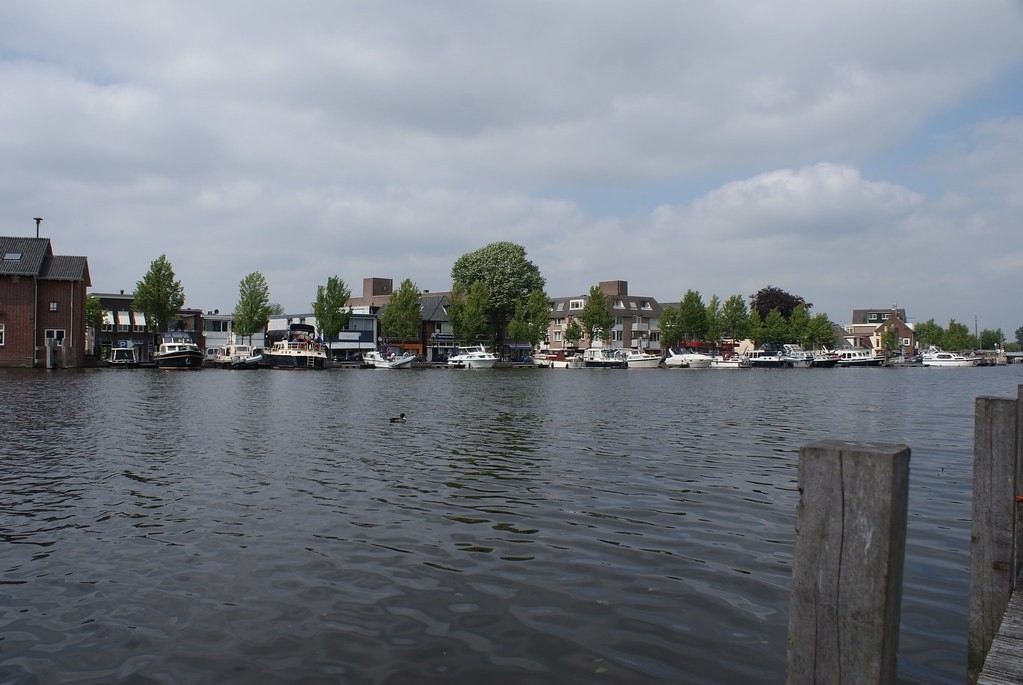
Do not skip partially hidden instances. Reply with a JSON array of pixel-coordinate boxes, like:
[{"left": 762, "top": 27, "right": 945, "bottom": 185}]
[
  {"left": 378, "top": 336, "right": 383, "bottom": 355},
  {"left": 104, "top": 318, "right": 111, "bottom": 358},
  {"left": 230, "top": 329, "right": 234, "bottom": 344}
]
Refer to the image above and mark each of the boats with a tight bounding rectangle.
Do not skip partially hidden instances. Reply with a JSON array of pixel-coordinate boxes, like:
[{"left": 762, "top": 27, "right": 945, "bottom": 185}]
[
  {"left": 922, "top": 346, "right": 984, "bottom": 367},
  {"left": 361, "top": 347, "right": 417, "bottom": 369},
  {"left": 527, "top": 344, "right": 888, "bottom": 369},
  {"left": 251, "top": 323, "right": 329, "bottom": 368},
  {"left": 446, "top": 343, "right": 502, "bottom": 368},
  {"left": 151, "top": 328, "right": 206, "bottom": 371},
  {"left": 213, "top": 330, "right": 263, "bottom": 371},
  {"left": 105, "top": 344, "right": 140, "bottom": 370}
]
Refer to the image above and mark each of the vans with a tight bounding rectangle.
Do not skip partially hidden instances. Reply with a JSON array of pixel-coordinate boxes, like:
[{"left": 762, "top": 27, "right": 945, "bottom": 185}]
[{"left": 205, "top": 347, "right": 219, "bottom": 361}]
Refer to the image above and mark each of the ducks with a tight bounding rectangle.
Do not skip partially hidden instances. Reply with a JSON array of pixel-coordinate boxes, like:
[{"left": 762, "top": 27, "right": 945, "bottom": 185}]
[{"left": 390, "top": 412, "right": 406, "bottom": 423}]
